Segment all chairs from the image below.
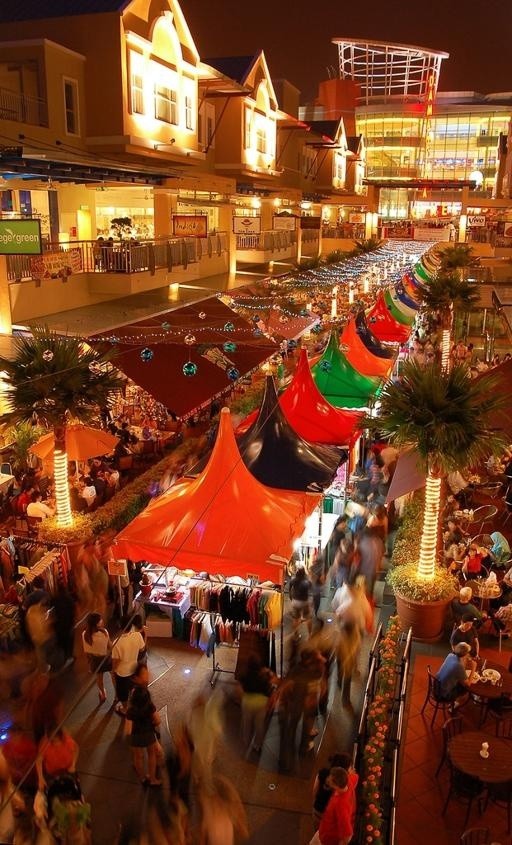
[6,403,135,531]
[419,484,512,845]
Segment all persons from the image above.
[1,313,512,845]
[93,237,140,272]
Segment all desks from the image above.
[134,577,199,640]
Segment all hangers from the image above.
[193,610,234,627]
[11,532,64,552]
